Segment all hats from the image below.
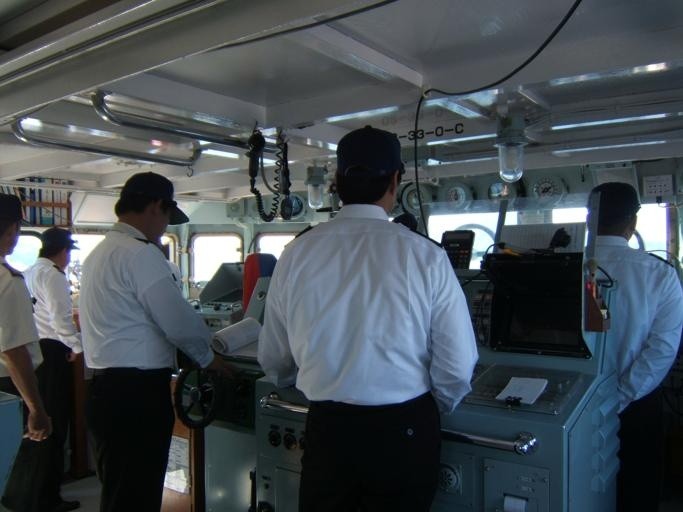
[40,226,79,250]
[588,183,640,219]
[0,194,32,227]
[121,171,189,225]
[338,125,404,174]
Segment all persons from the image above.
[584,182,683,512]
[0,193,54,511]
[257,126,478,509]
[2,229,84,511]
[79,172,222,512]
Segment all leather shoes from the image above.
[57,500,80,512]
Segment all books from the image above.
[214,316,262,361]
